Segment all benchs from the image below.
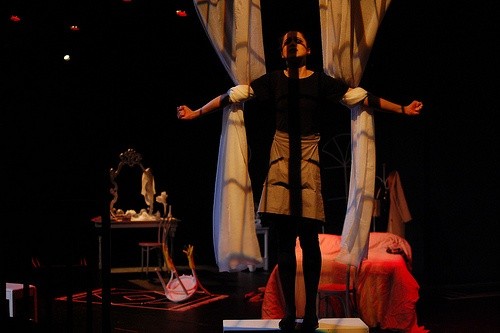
[223,317,368,333]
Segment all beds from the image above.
[262,233,416,328]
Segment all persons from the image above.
[176,27,422,331]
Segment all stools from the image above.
[6,283,39,324]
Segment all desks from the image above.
[92,216,178,276]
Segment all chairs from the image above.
[158,245,217,302]
[317,260,359,317]
[138,217,175,279]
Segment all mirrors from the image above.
[109,149,156,217]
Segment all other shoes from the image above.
[278,314,320,332]
